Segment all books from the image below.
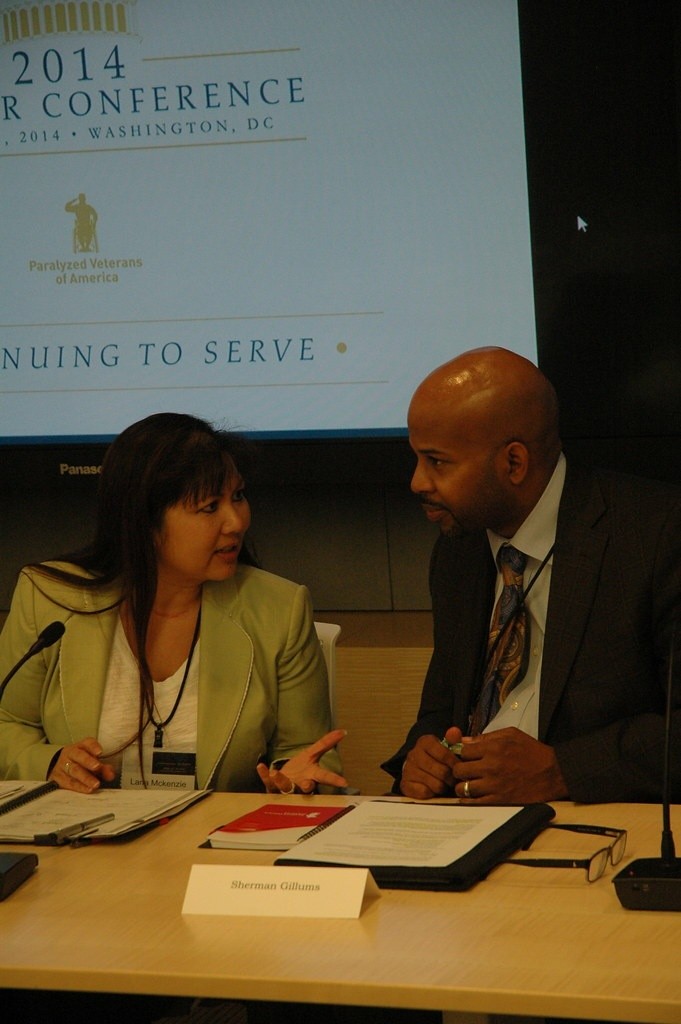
[207,804,355,851]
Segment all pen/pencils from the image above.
[47,813,115,845]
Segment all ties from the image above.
[467,543,529,739]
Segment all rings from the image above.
[64,761,73,774]
[280,782,295,795]
[464,781,471,797]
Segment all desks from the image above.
[0,782,681,1024]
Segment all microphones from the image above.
[0,622,66,702]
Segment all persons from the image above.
[0,413,351,795]
[381,346,681,805]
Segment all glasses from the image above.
[482,824,628,883]
